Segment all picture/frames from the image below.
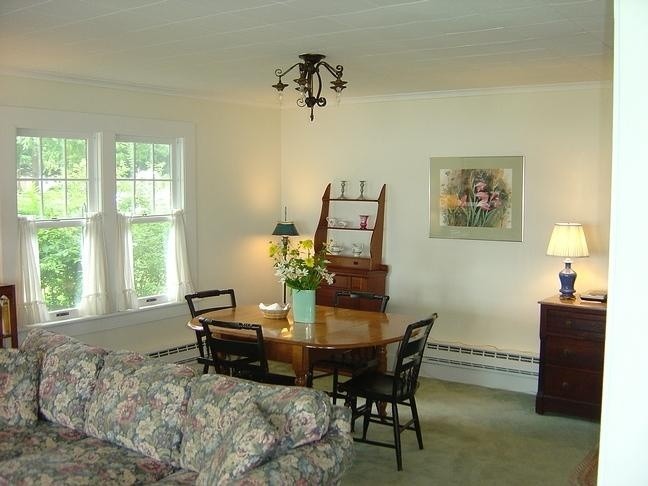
[429,156,522,243]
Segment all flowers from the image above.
[267,238,335,290]
[439,168,510,228]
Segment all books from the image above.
[579,289,607,301]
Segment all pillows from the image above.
[182,376,330,473]
[198,406,279,486]
[0,348,40,430]
[83,352,194,468]
[39,343,106,433]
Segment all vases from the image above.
[292,289,316,323]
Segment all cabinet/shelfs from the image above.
[306,182,387,377]
[535,294,606,424]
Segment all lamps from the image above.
[547,222,590,299]
[271,206,300,304]
[273,53,354,122]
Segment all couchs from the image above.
[0,332,353,485]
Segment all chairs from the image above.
[198,317,296,387]
[185,289,258,373]
[337,313,438,472]
[308,291,389,419]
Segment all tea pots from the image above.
[326,216,338,227]
